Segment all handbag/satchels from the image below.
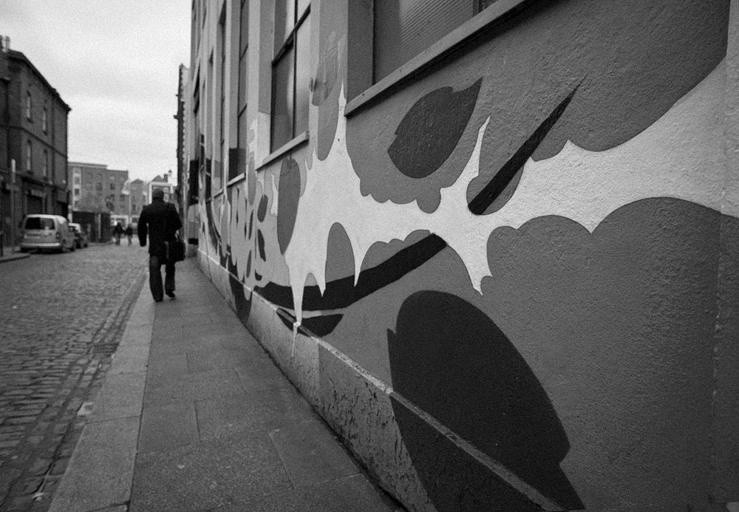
[164,235,188,263]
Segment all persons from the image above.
[112,222,125,245]
[137,188,183,303]
[125,225,133,245]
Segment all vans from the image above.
[18,214,77,254]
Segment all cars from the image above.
[68,223,88,249]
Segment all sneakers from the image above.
[154,297,164,303]
[165,289,176,300]
